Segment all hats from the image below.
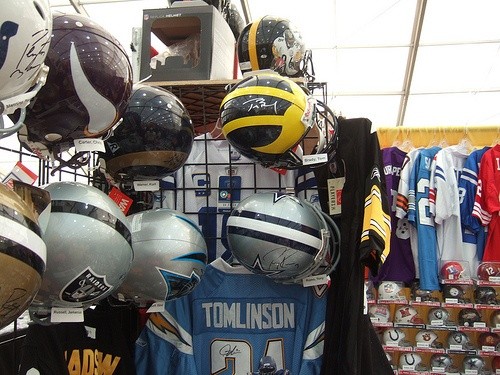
[218,71,340,171]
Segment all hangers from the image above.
[377,128,500,152]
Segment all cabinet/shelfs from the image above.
[367,277,500,375]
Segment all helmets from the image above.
[362,260,500,375]
[9,15,134,156]
[114,209,209,303]
[0,180,51,329]
[97,85,194,182]
[31,183,135,309]
[237,14,315,88]
[0,0,53,139]
[224,191,342,283]
